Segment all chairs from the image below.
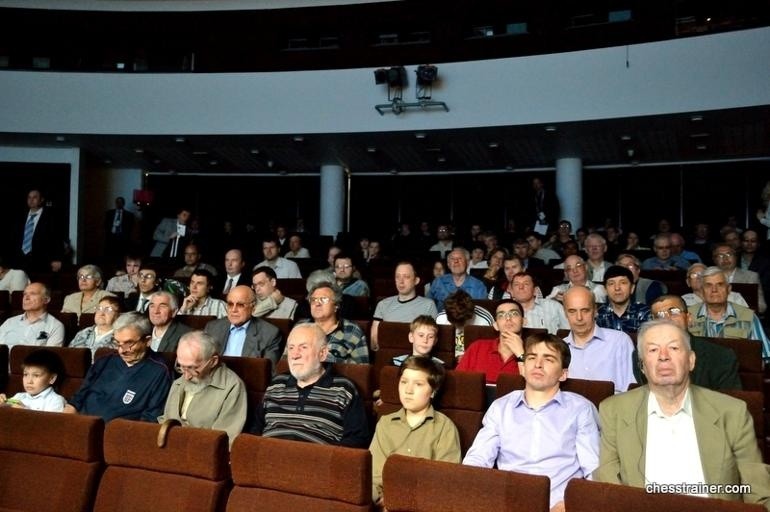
[290,257,316,276]
[218,356,272,436]
[274,360,374,443]
[292,317,370,342]
[0,405,105,512]
[276,278,309,315]
[627,381,765,464]
[526,268,564,297]
[376,364,486,463]
[93,346,182,386]
[373,321,457,369]
[93,417,227,510]
[641,268,681,296]
[693,336,765,392]
[494,372,617,413]
[337,293,377,317]
[223,431,373,512]
[175,314,217,332]
[10,289,62,315]
[6,344,91,402]
[12,310,77,347]
[463,324,548,354]
[381,452,550,511]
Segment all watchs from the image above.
[512,355,527,363]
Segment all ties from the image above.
[139,299,149,313]
[172,234,177,258]
[224,278,233,294]
[21,213,38,254]
[116,211,121,231]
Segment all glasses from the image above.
[174,358,211,377]
[110,340,146,350]
[335,264,352,268]
[566,264,586,271]
[226,302,254,308]
[743,238,758,244]
[252,280,269,290]
[311,298,337,305]
[438,229,449,232]
[79,275,98,280]
[559,224,570,228]
[94,306,119,313]
[715,253,733,259]
[655,308,684,318]
[655,245,672,251]
[496,310,524,317]
[138,273,157,280]
[621,263,638,268]
[688,272,701,280]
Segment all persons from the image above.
[632,295,741,388]
[645,237,684,273]
[492,253,543,298]
[437,292,496,359]
[425,259,444,299]
[736,233,767,283]
[421,223,433,240]
[553,221,582,259]
[287,234,309,260]
[358,240,370,258]
[98,198,135,260]
[251,240,303,281]
[621,230,648,252]
[427,248,489,315]
[2,264,31,304]
[613,256,667,307]
[6,191,61,280]
[509,240,544,277]
[142,293,194,354]
[70,312,174,421]
[503,274,567,330]
[370,261,438,353]
[323,252,370,299]
[545,253,606,302]
[157,330,250,450]
[106,258,143,300]
[277,283,369,369]
[274,227,289,254]
[687,222,713,263]
[576,227,588,251]
[118,268,162,319]
[67,297,120,352]
[217,222,237,256]
[0,284,64,349]
[395,221,412,243]
[597,319,770,510]
[523,174,559,239]
[460,336,602,511]
[366,358,462,510]
[606,226,619,249]
[211,249,251,297]
[581,234,612,282]
[685,267,769,368]
[250,323,365,451]
[463,243,487,281]
[667,232,699,269]
[364,244,383,274]
[650,219,669,241]
[596,267,649,335]
[374,314,446,408]
[206,286,279,379]
[151,212,190,257]
[528,234,559,265]
[60,266,115,316]
[422,262,445,298]
[485,236,509,255]
[454,300,529,386]
[718,229,744,265]
[307,246,361,295]
[2,352,66,410]
[468,225,481,240]
[710,243,765,313]
[178,269,227,321]
[556,286,635,394]
[478,248,507,296]
[430,226,454,256]
[250,268,298,320]
[175,246,221,289]
[681,262,750,309]
